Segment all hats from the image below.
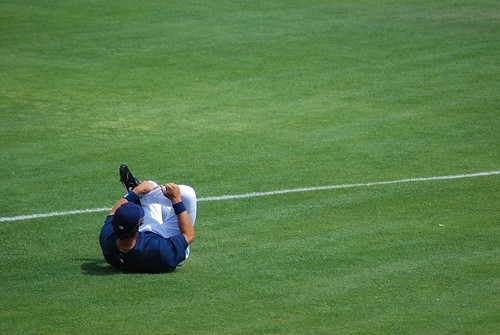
[114,202,145,227]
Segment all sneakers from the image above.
[120,164,142,199]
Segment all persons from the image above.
[99,164,197,274]
